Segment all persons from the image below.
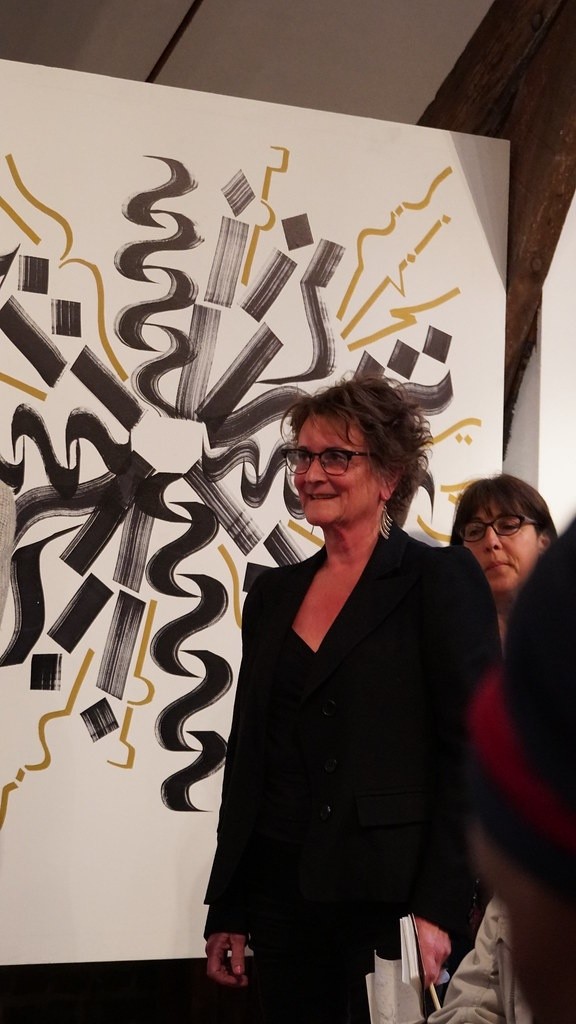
[427,472,576,1024]
[203,375,502,1024]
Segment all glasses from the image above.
[281,448,376,476]
[458,514,539,542]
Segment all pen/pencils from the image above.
[425,974,442,1012]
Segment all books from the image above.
[364,914,443,1024]
